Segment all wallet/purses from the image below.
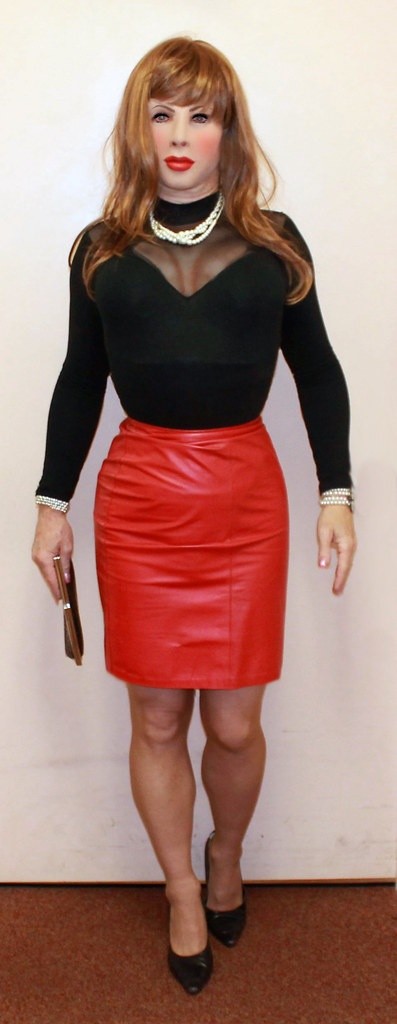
[53,556,84,666]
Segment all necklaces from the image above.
[149,193,226,245]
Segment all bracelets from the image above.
[319,488,354,512]
[35,494,69,514]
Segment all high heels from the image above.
[205,830,246,948]
[168,937,213,996]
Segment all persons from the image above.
[31,38,355,997]
